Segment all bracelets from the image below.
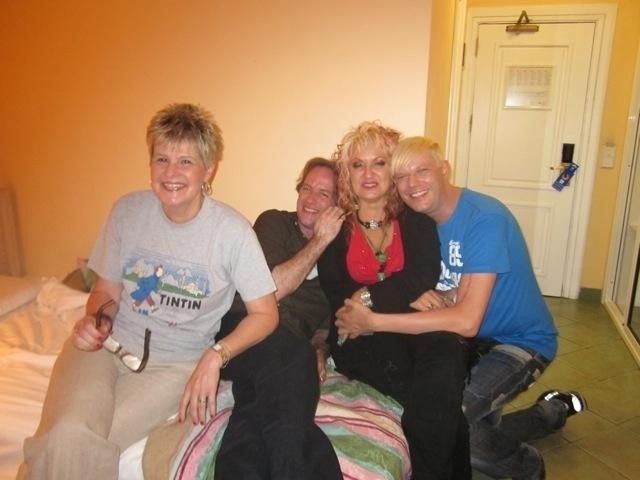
[209,342,233,368]
[358,284,374,311]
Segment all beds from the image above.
[0,182,413,480]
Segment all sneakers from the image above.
[536,388,588,419]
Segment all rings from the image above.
[428,304,433,309]
[443,295,448,301]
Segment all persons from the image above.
[14,101,280,480]
[334,132,590,480]
[317,117,472,480]
[215,153,347,480]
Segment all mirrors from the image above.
[626,246,640,344]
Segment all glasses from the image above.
[96,299,151,372]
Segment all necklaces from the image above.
[360,224,392,282]
[355,207,392,228]
